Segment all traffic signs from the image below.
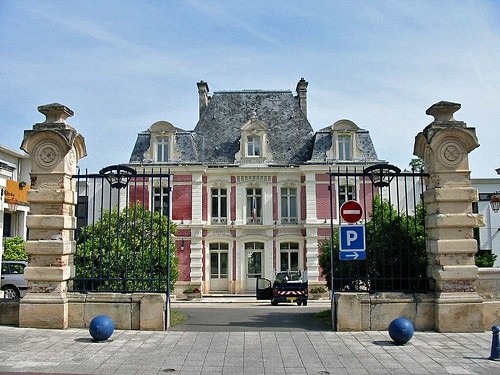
[338,225,367,260]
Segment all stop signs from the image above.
[339,200,363,223]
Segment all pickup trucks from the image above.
[255,270,309,307]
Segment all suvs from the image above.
[0,259,28,301]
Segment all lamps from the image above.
[181,238,184,251]
[487,191,500,212]
[19,182,26,188]
